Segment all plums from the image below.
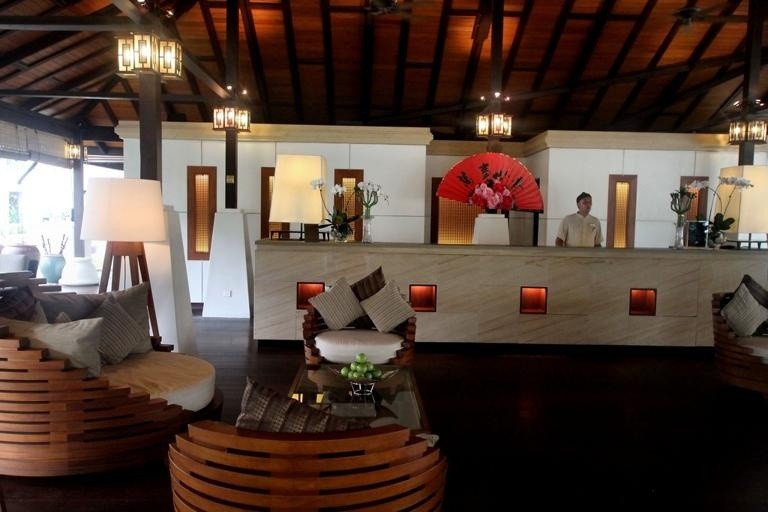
[339,353,383,379]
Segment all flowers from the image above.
[669,180,708,226]
[708,177,754,243]
[356,182,390,217]
[470,178,515,214]
[312,179,358,239]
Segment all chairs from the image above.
[0,272,223,476]
[170,419,448,512]
[302,296,416,365]
[711,291,766,393]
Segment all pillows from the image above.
[309,267,415,333]
[2,279,151,379]
[718,275,768,340]
[236,377,368,433]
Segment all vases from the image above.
[708,232,727,249]
[330,226,353,243]
[472,214,510,246]
[674,225,684,248]
[360,218,374,242]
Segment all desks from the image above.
[288,364,432,438]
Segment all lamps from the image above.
[80,179,165,340]
[729,98,767,145]
[476,91,516,139]
[116,0,183,80]
[213,84,251,132]
[269,154,328,242]
[63,142,88,162]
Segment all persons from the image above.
[555,192,604,247]
